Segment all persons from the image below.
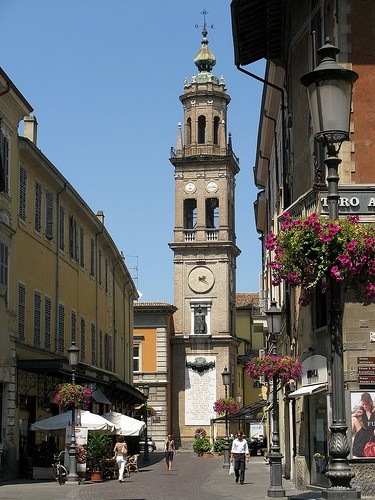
[113,435,127,482]
[234,433,248,470]
[230,430,249,485]
[352,392,375,458]
[164,435,175,471]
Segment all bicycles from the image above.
[52,451,68,486]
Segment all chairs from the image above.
[125,453,139,477]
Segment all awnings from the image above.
[19,355,149,404]
[288,381,327,397]
[100,411,147,436]
[30,409,122,434]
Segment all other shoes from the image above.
[235,480,239,484]
[118,479,124,483]
[240,481,244,485]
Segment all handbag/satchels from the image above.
[122,446,127,454]
[229,462,235,477]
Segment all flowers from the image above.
[51,383,92,409]
[213,398,240,415]
[245,355,304,386]
[265,210,375,305]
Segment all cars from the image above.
[138,436,155,453]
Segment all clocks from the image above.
[188,266,216,294]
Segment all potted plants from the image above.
[83,428,115,482]
[193,438,211,458]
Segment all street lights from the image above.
[263,297,286,498]
[139,384,150,461]
[66,338,80,482]
[220,365,231,469]
[300,34,358,492]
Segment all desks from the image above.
[100,457,114,463]
